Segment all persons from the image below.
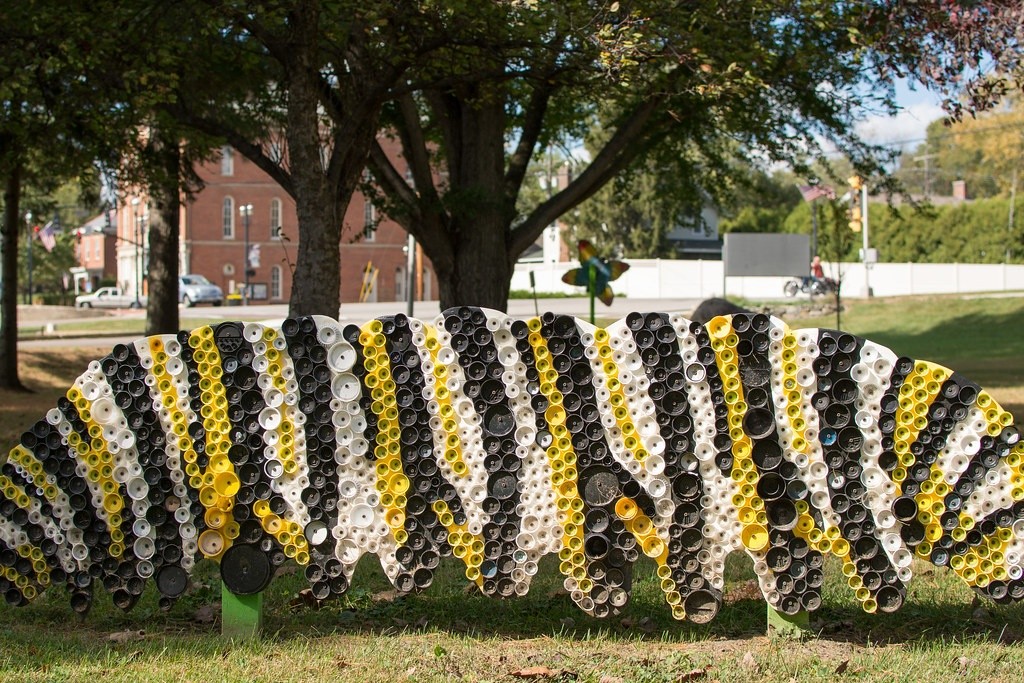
[810,256,825,290]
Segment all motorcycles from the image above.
[783,274,838,299]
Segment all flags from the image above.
[38,220,56,253]
[795,183,835,202]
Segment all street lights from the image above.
[131,195,149,307]
[25,208,32,305]
[238,204,255,306]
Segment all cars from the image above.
[74,285,148,310]
[179,274,224,307]
